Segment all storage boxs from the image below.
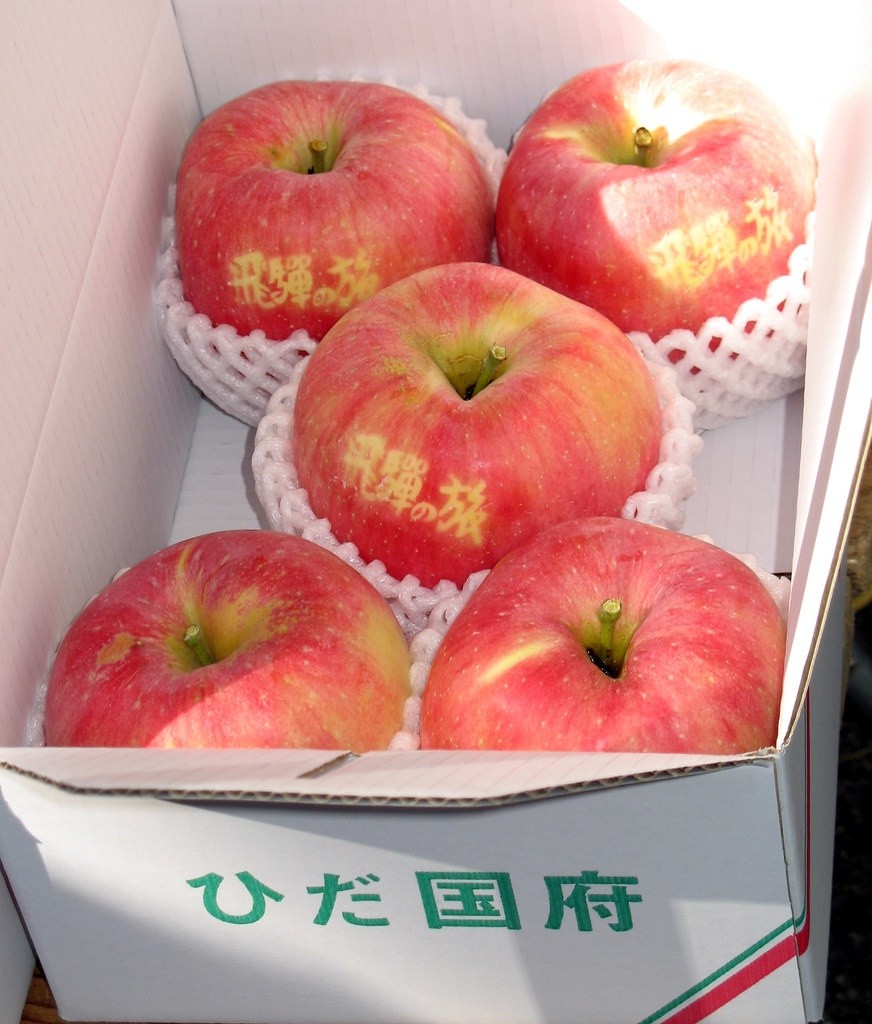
[0,0,872,1024]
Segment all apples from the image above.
[45,60,815,755]
